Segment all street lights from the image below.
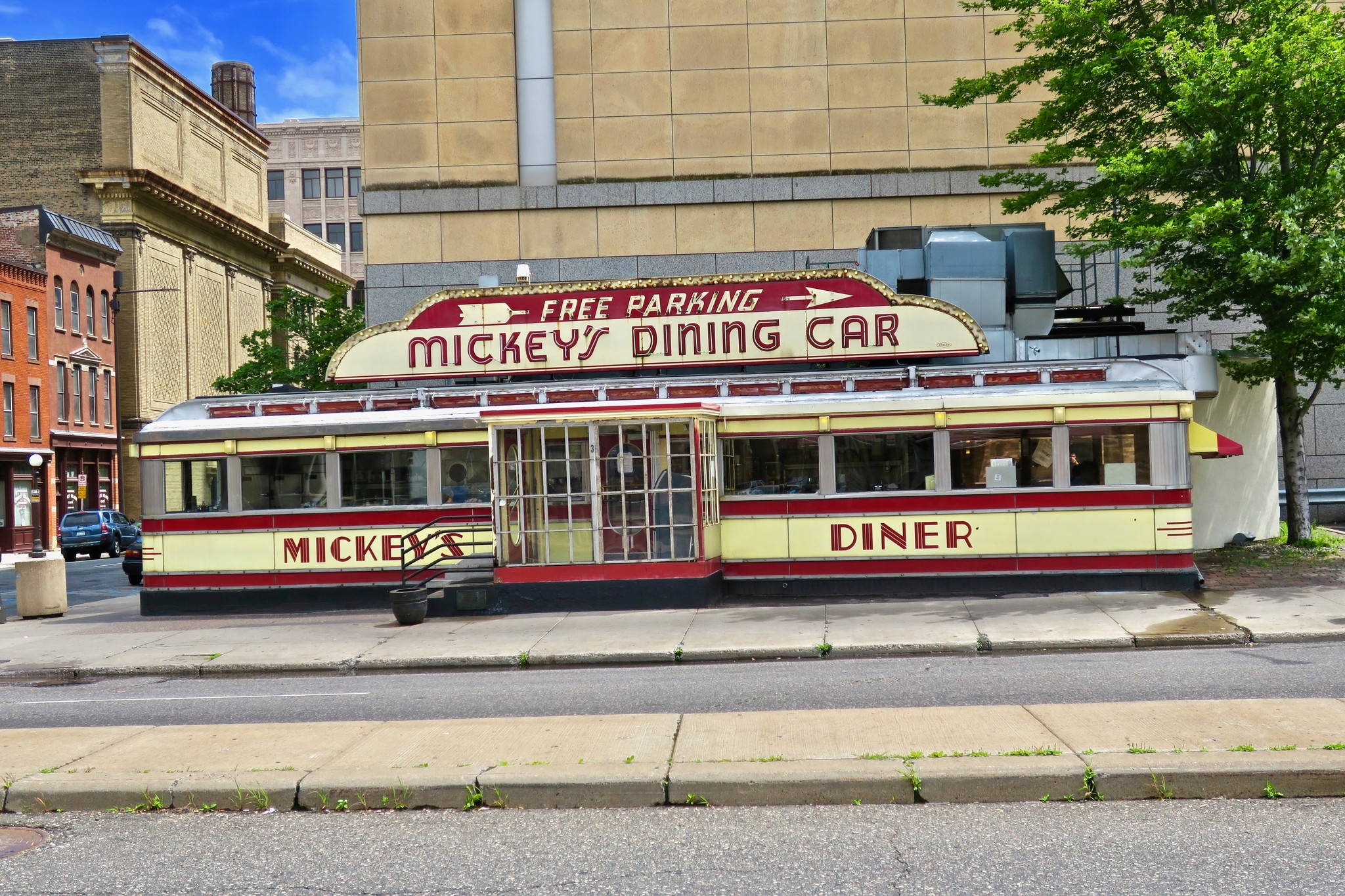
[28,454,47,558]
[112,288,181,514]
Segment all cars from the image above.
[122,518,143,586]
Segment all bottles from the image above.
[202,501,205,512]
[362,498,366,506]
[206,504,208,512]
[209,504,212,512]
[374,495,377,505]
[187,509,191,512]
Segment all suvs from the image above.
[57,508,142,562]
